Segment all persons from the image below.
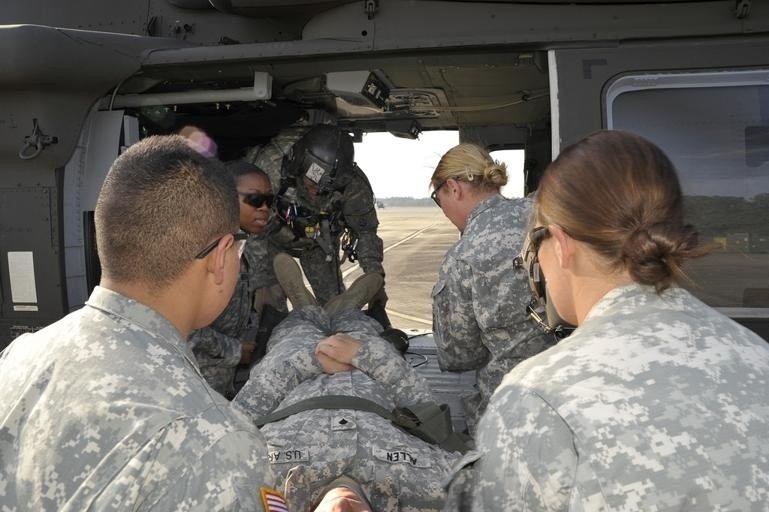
[427,143,565,439]
[229,252,464,512]
[472,125,769,512]
[0,136,272,512]
[189,163,281,393]
[247,118,387,310]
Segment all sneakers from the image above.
[274,252,316,309]
[323,271,383,315]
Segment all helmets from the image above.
[288,125,354,192]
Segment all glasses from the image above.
[195,226,251,259]
[236,192,275,209]
[430,180,450,208]
[530,225,551,253]
